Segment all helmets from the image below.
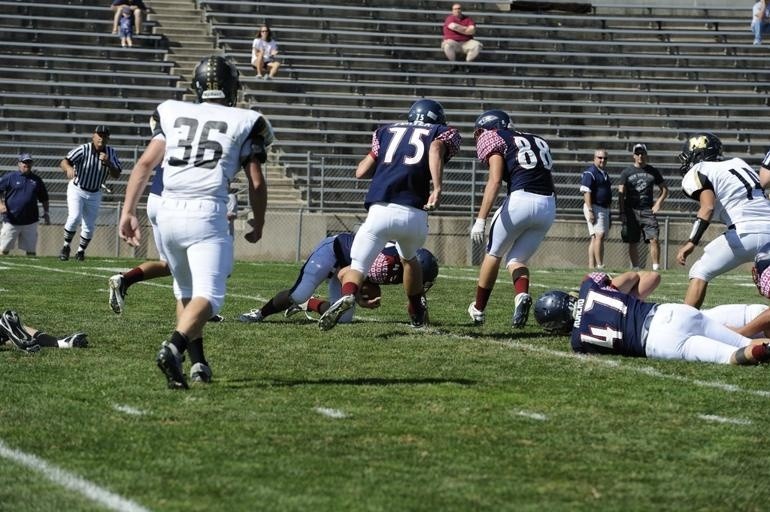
[681,132,725,169]
[473,109,515,136]
[189,54,242,103]
[531,290,578,334]
[416,246,438,287]
[405,98,447,128]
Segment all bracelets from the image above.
[44,211,50,216]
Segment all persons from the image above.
[119,8,133,48]
[441,4,483,73]
[751,0,770,45]
[536,270,770,365]
[251,26,280,79]
[59,125,122,261]
[677,132,770,308]
[317,100,462,331]
[468,111,557,328]
[110,0,146,35]
[241,233,438,324]
[0,153,51,256]
[0,311,90,354]
[109,160,237,324]
[117,55,273,390]
[580,149,612,269]
[618,143,667,271]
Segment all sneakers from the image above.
[155,339,189,391]
[107,274,128,315]
[0,310,41,352]
[467,298,484,326]
[61,332,89,348]
[317,291,355,333]
[73,251,86,261]
[510,292,535,330]
[283,294,314,319]
[239,308,265,323]
[206,314,225,322]
[59,246,70,260]
[189,362,213,384]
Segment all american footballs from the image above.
[360,283,381,305]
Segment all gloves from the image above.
[468,217,488,244]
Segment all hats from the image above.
[94,125,111,135]
[632,142,648,155]
[17,153,34,163]
